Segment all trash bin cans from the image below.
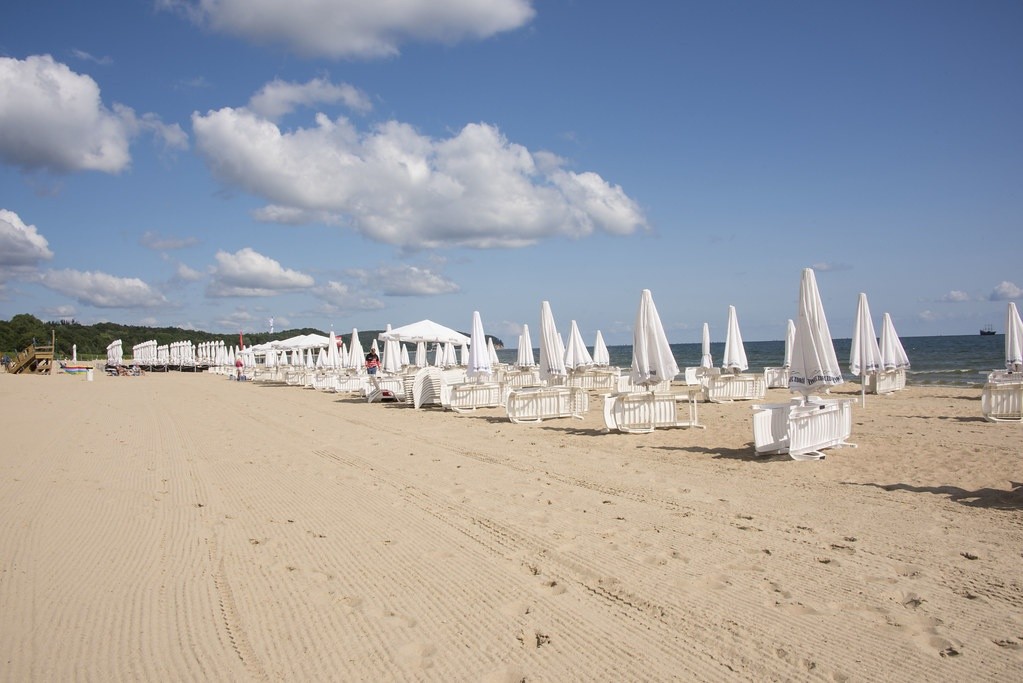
[86,367,93,381]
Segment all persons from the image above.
[364,346,381,375]
[236,358,244,376]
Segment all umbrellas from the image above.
[782,319,797,373]
[564,319,594,371]
[401,344,410,366]
[517,323,535,367]
[628,289,680,391]
[105,339,225,367]
[538,301,568,381]
[215,344,315,368]
[372,339,381,362]
[558,332,566,360]
[237,333,342,356]
[416,342,470,368]
[879,312,911,375]
[720,304,749,377]
[466,311,493,378]
[1005,302,1023,372]
[788,268,845,403]
[487,337,499,366]
[592,330,610,367]
[378,321,472,346]
[700,322,714,378]
[317,328,365,371]
[380,324,402,373]
[848,292,885,409]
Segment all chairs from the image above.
[105,363,1023,460]
